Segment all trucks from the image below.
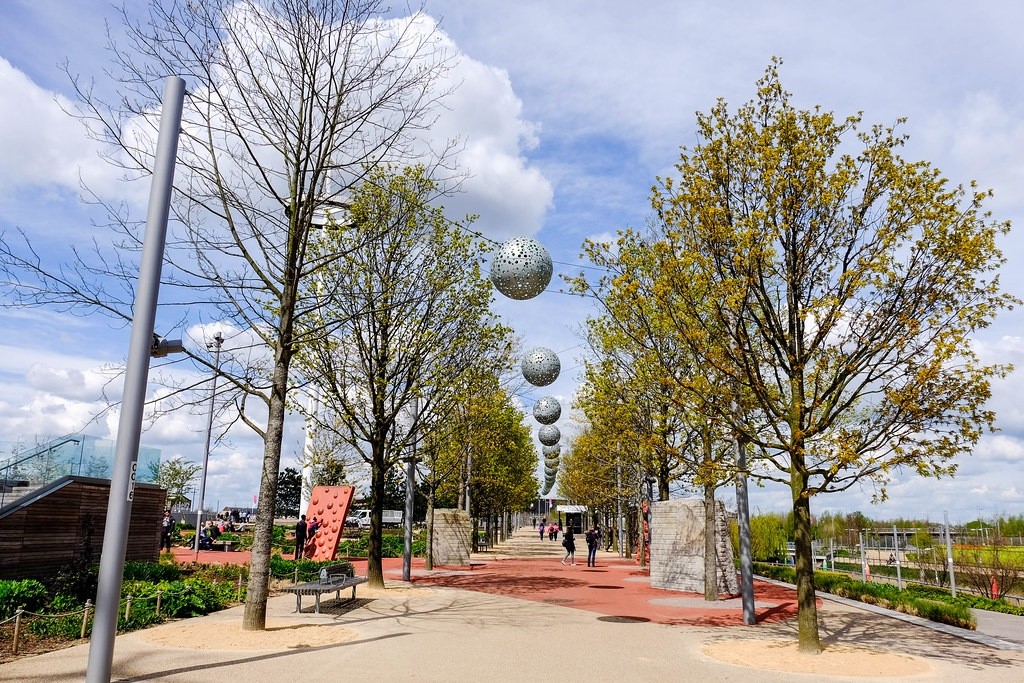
[347,510,403,529]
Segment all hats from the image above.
[313,517,317,520]
[590,526,594,530]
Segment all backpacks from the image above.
[549,527,554,532]
[562,540,566,547]
[540,526,543,530]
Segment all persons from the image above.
[585,527,602,568]
[160,510,175,553]
[217,509,250,532]
[532,517,536,529]
[542,517,547,525]
[569,519,573,526]
[185,521,219,551]
[606,526,614,552]
[295,515,321,561]
[538,523,559,542]
[561,527,576,566]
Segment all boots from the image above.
[588,556,590,567]
[592,556,594,567]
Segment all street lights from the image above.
[194,331,225,552]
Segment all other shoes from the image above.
[571,564,576,566]
[561,560,565,565]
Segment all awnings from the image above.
[556,505,588,527]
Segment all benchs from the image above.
[278,576,369,614]
[187,539,239,553]
[477,543,490,552]
